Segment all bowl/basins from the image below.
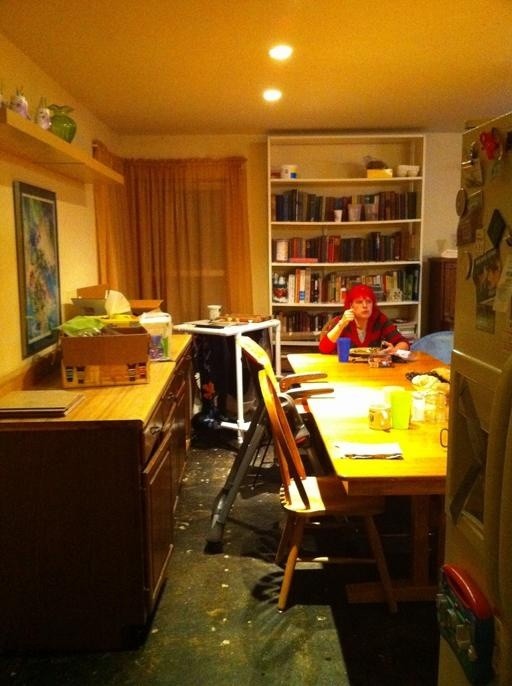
[411,374,441,393]
[437,381,449,396]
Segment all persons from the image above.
[319,284,410,356]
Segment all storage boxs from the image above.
[70,284,165,316]
[59,326,153,390]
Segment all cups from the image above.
[387,390,413,431]
[207,305,222,319]
[423,392,446,426]
[368,404,391,431]
[333,209,343,222]
[336,337,351,362]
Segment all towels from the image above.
[334,441,404,460]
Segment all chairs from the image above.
[257,368,399,611]
[204,335,336,544]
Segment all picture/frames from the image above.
[444,350,507,558]
[14,180,62,360]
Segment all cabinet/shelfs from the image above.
[267,133,426,359]
[171,317,282,451]
[1,332,193,644]
[428,257,458,332]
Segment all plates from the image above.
[348,347,384,357]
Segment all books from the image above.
[0,390,86,418]
[271,188,419,340]
